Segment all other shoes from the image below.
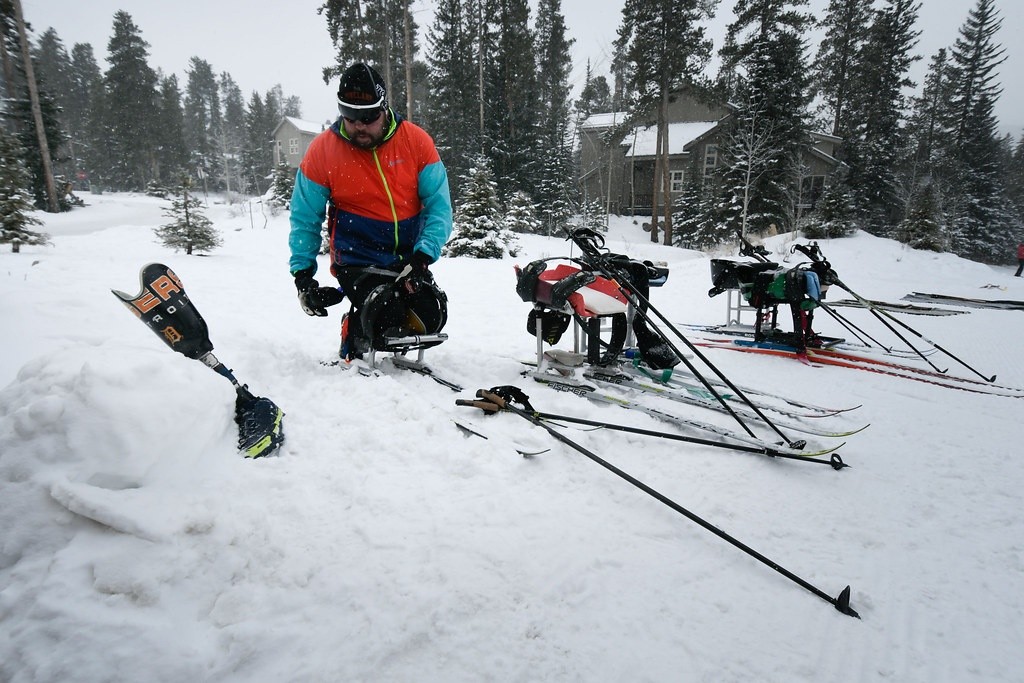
[234,395,285,459]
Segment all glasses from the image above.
[336,104,382,126]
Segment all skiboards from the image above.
[673,322,1024,399]
[519,354,873,458]
[317,349,612,457]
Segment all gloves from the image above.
[394,254,432,293]
[291,272,344,317]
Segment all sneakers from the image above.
[337,312,365,365]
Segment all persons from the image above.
[1015,241,1024,277]
[288,63,453,364]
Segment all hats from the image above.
[337,63,386,106]
